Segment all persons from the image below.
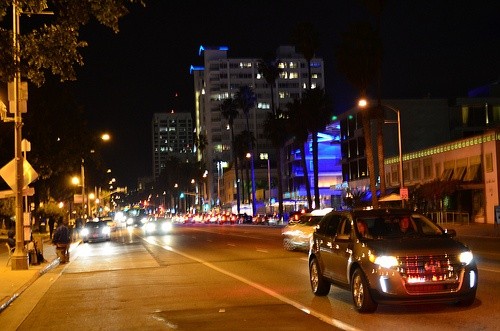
[391,217,414,235]
[7,231,48,265]
[356,220,375,238]
[48,215,55,239]
[52,217,70,264]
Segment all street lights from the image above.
[358,93,404,208]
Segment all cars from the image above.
[77,205,293,244]
[282,206,335,250]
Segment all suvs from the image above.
[309,208,479,311]
